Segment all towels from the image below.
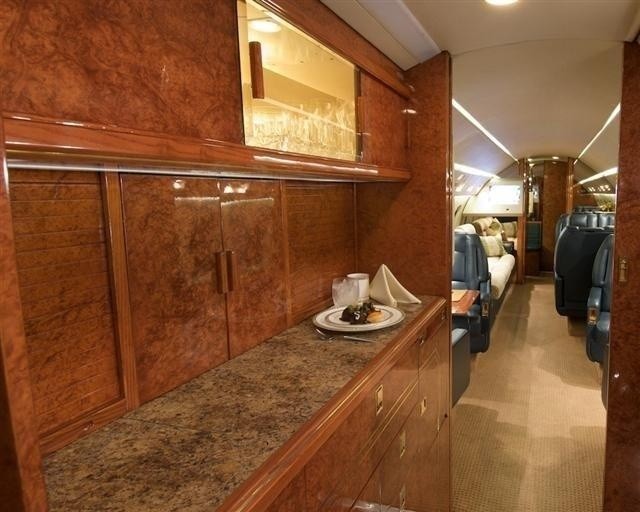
[368,264,422,308]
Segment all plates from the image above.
[312,305,406,332]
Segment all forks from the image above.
[313,328,376,343]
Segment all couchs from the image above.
[452,234,490,352]
[471,217,518,255]
[456,225,515,317]
[567,213,614,227]
[553,226,614,316]
[586,235,614,361]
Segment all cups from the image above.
[347,273,369,300]
[331,277,359,307]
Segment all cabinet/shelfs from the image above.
[1,0,454,511]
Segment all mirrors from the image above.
[462,214,518,255]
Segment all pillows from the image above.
[487,230,503,241]
[490,218,503,230]
[480,235,506,257]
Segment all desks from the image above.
[452,290,479,313]
